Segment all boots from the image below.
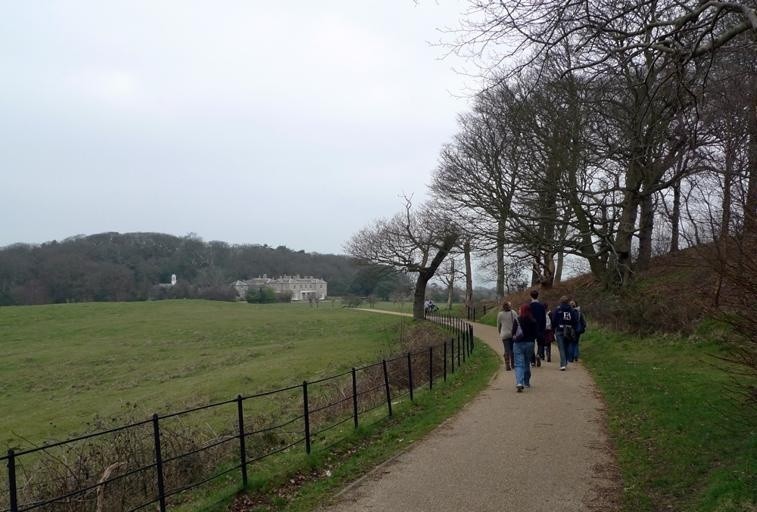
[504,351,514,370]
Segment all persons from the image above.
[496,289,585,371]
[511,304,537,392]
[424,298,433,319]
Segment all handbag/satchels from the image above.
[546,311,553,330]
[577,312,585,333]
[512,317,524,341]
[564,325,576,342]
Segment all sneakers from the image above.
[531,352,578,370]
[516,383,530,392]
